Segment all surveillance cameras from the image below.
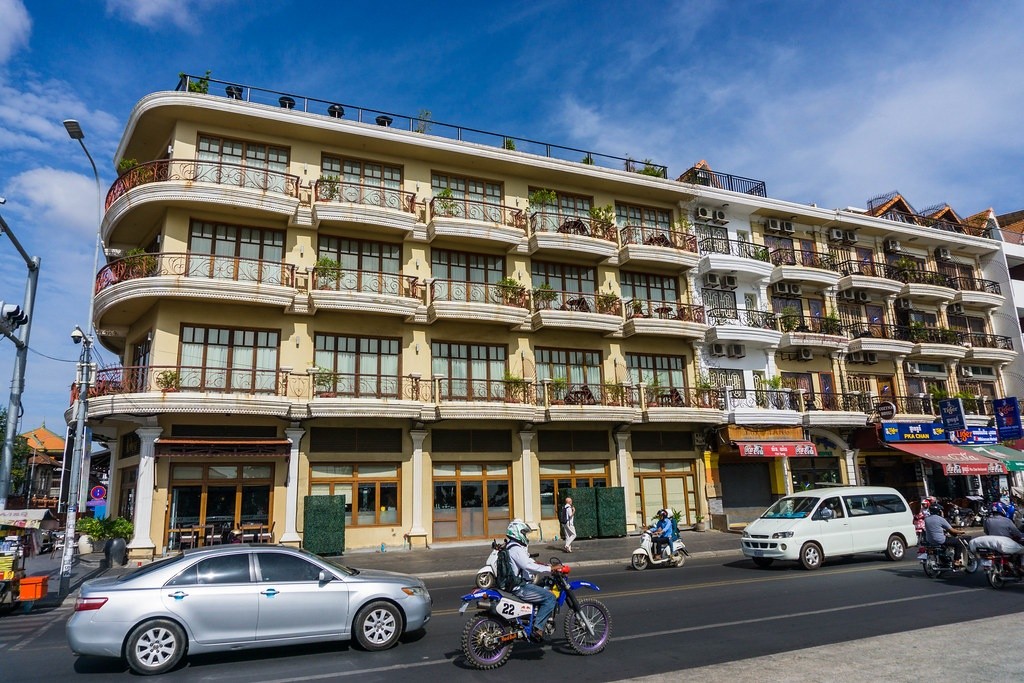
[71,330,82,343]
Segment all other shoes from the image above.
[564,544,572,553]
[1014,566,1024,576]
[954,561,965,567]
[670,554,674,560]
[652,552,661,561]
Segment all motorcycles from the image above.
[916,531,980,579]
[976,547,1024,588]
[461,556,613,671]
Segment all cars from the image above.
[0,529,79,558]
[65,542,433,676]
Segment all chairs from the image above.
[177,524,196,551]
[241,524,262,544]
[206,525,223,547]
[258,521,276,543]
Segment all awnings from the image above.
[886,443,1024,476]
[732,440,819,458]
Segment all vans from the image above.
[740,486,918,571]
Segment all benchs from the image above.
[127,547,155,562]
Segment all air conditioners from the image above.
[728,344,746,357]
[779,222,795,235]
[843,231,859,245]
[849,352,864,363]
[884,239,902,253]
[854,291,872,304]
[721,275,738,290]
[713,211,730,224]
[934,248,951,261]
[948,303,965,316]
[839,289,855,301]
[896,298,913,310]
[710,343,726,358]
[829,229,843,241]
[863,352,879,364]
[958,366,974,377]
[694,207,714,220]
[797,348,814,360]
[904,361,920,375]
[703,271,720,285]
[773,282,789,295]
[765,219,781,234]
[786,284,803,297]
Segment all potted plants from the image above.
[312,255,345,291]
[75,514,134,553]
[643,373,665,408]
[548,377,569,405]
[309,364,347,399]
[587,203,617,241]
[631,297,645,318]
[156,369,183,392]
[494,276,527,307]
[123,248,159,278]
[695,383,713,408]
[499,368,529,404]
[533,281,558,310]
[317,172,341,202]
[601,380,625,406]
[528,188,558,232]
[436,187,461,217]
[597,290,620,315]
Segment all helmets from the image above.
[657,509,667,521]
[665,509,674,518]
[990,501,1006,517]
[921,499,930,508]
[929,503,942,515]
[506,521,532,546]
[926,496,936,505]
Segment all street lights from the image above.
[61,118,102,342]
[57,324,91,598]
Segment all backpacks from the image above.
[496,542,523,593]
[558,505,572,524]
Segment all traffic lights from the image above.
[0,298,29,335]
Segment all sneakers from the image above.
[532,627,551,643]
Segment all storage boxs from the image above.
[0,555,19,573]
[10,546,18,551]
[4,571,15,579]
[5,535,19,540]
[5,552,12,556]
[19,576,49,599]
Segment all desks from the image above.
[566,300,584,311]
[657,394,675,407]
[571,390,590,405]
[240,525,270,531]
[169,528,203,532]
[654,307,673,319]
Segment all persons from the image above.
[818,502,832,519]
[920,499,931,517]
[926,496,944,517]
[500,519,564,646]
[665,509,680,559]
[562,498,577,553]
[984,502,1024,573]
[925,504,966,567]
[645,510,673,559]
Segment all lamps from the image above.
[296,336,300,346]
[416,344,420,353]
[304,162,308,171]
[517,195,520,202]
[167,144,173,153]
[300,245,304,254]
[148,331,153,341]
[415,258,420,266]
[610,279,613,287]
[522,350,525,359]
[157,234,161,243]
[518,269,522,277]
[417,180,420,187]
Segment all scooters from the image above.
[632,529,690,572]
[914,499,992,541]
[474,539,501,590]
